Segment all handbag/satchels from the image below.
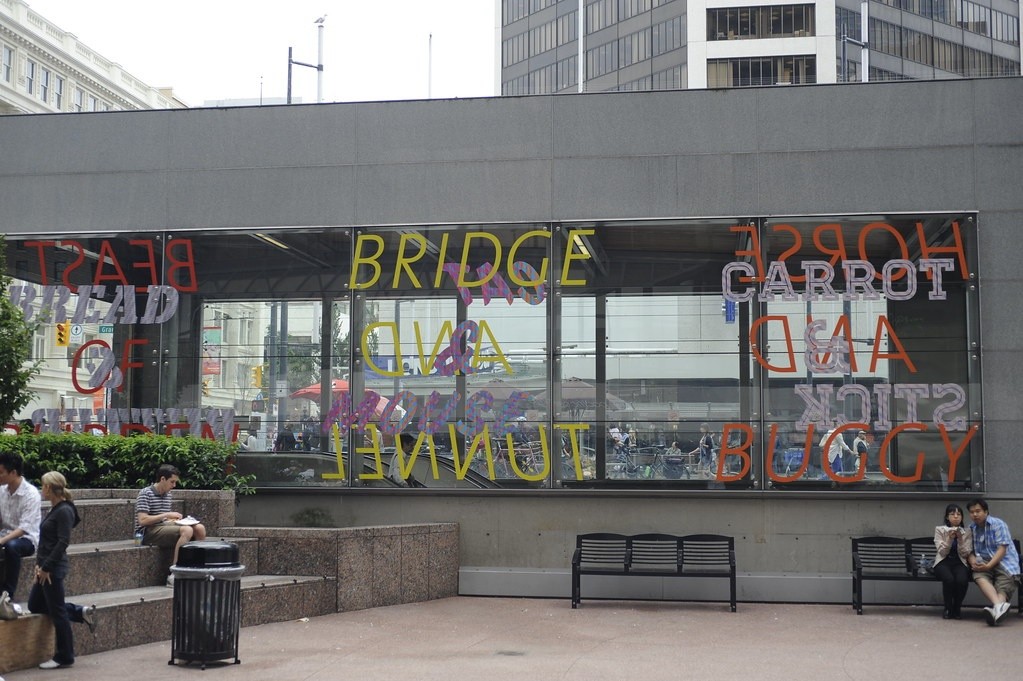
[918,552,931,575]
[709,449,717,462]
[0,591,18,620]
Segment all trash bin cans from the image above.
[167,542,246,668]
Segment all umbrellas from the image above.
[467,378,531,417]
[289,378,368,406]
[535,377,625,434]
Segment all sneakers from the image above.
[983,606,995,626]
[993,601,1011,623]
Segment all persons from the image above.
[387,433,417,487]
[689,423,713,479]
[0,451,41,620]
[606,424,643,454]
[768,425,783,475]
[967,498,1021,625]
[295,406,384,450]
[665,441,682,455]
[853,431,869,478]
[239,430,257,451]
[27,471,96,669]
[933,504,976,619]
[134,464,206,588]
[818,421,857,474]
[561,436,570,459]
[276,420,295,451]
[505,417,533,473]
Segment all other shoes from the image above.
[864,476,869,479]
[81,605,98,633]
[165,575,174,587]
[943,609,951,619]
[952,612,961,620]
[39,658,74,670]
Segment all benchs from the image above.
[850,537,1023,615]
[572,533,737,612]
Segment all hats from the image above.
[768,425,773,428]
[753,424,758,428]
[859,430,867,434]
